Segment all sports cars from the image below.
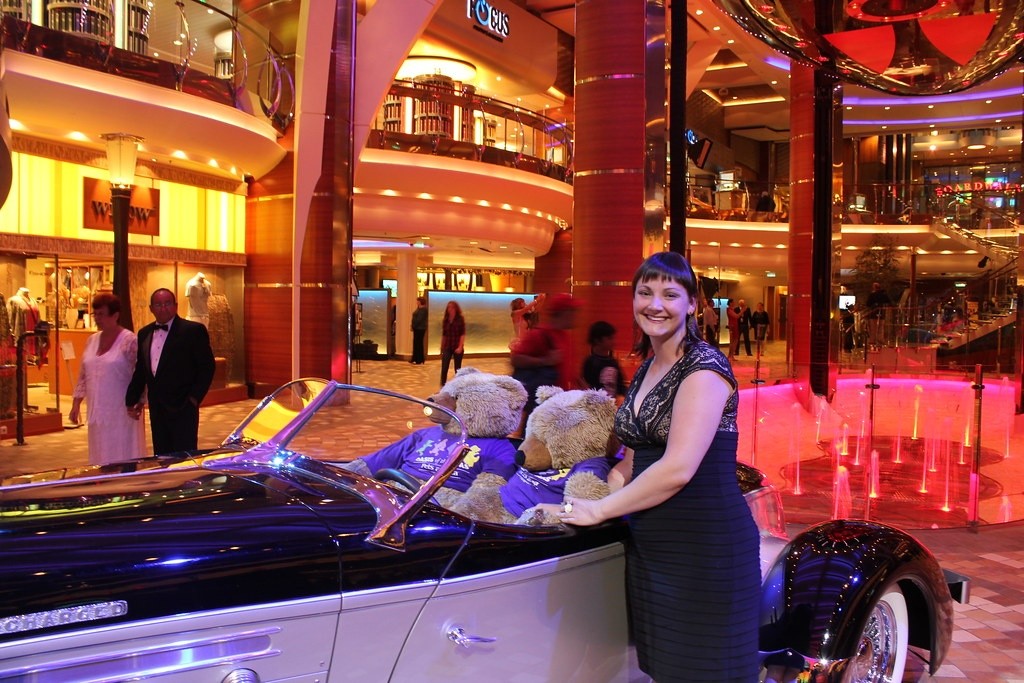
[1,372,973,683]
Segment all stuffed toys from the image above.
[457,386,624,527]
[340,366,528,518]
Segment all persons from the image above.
[557,252,762,683]
[439,301,466,387]
[724,298,769,361]
[756,191,776,213]
[703,300,720,350]
[125,288,216,459]
[8,287,41,353]
[184,272,211,328]
[69,293,146,467]
[409,295,429,365]
[509,292,631,436]
[844,304,857,353]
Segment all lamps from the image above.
[685,137,714,172]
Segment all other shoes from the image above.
[746,353,752,356]
[734,352,739,356]
[761,351,764,356]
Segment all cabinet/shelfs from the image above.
[44,259,148,392]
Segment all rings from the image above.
[565,504,572,513]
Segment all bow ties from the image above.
[152,324,169,332]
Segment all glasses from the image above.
[152,301,176,310]
[89,311,111,318]
